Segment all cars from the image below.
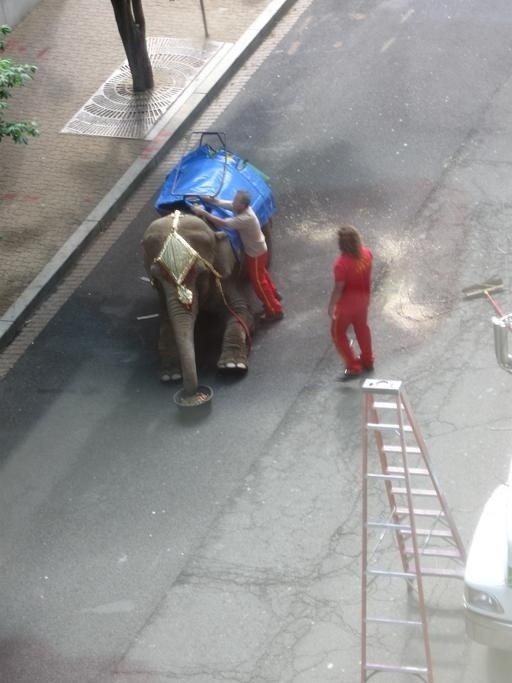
[462,480,511,650]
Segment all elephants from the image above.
[139,148,273,398]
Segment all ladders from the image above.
[361,378,467,683]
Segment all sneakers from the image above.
[259,292,283,321]
[339,356,374,379]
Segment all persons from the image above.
[191,191,284,322]
[328,226,373,380]
